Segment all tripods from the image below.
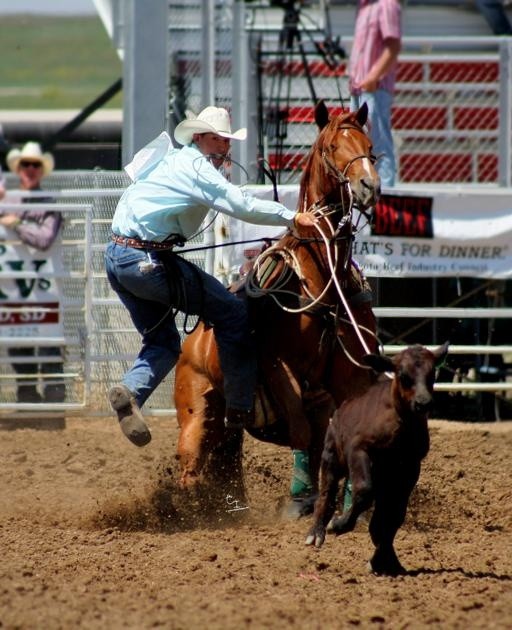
[264,11,333,183]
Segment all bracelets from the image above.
[11,220,21,231]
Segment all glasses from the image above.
[20,161,42,169]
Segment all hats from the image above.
[173,105,248,148]
[6,141,55,177]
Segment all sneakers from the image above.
[223,406,245,430]
[108,384,152,447]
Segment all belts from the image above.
[111,231,174,252]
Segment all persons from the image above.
[104,106,320,446]
[2,140,68,403]
[347,1,404,187]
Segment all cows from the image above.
[305,340,448,577]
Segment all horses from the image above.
[175,98,379,515]
